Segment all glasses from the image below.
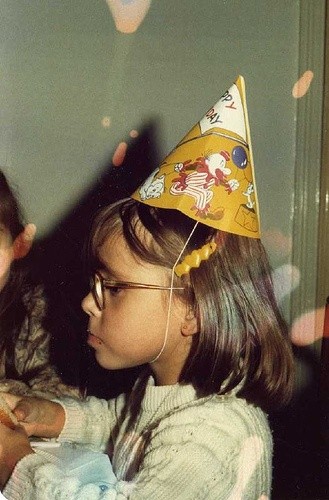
[90,270,187,311]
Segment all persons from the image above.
[0,75,295,500]
[0,169,85,412]
